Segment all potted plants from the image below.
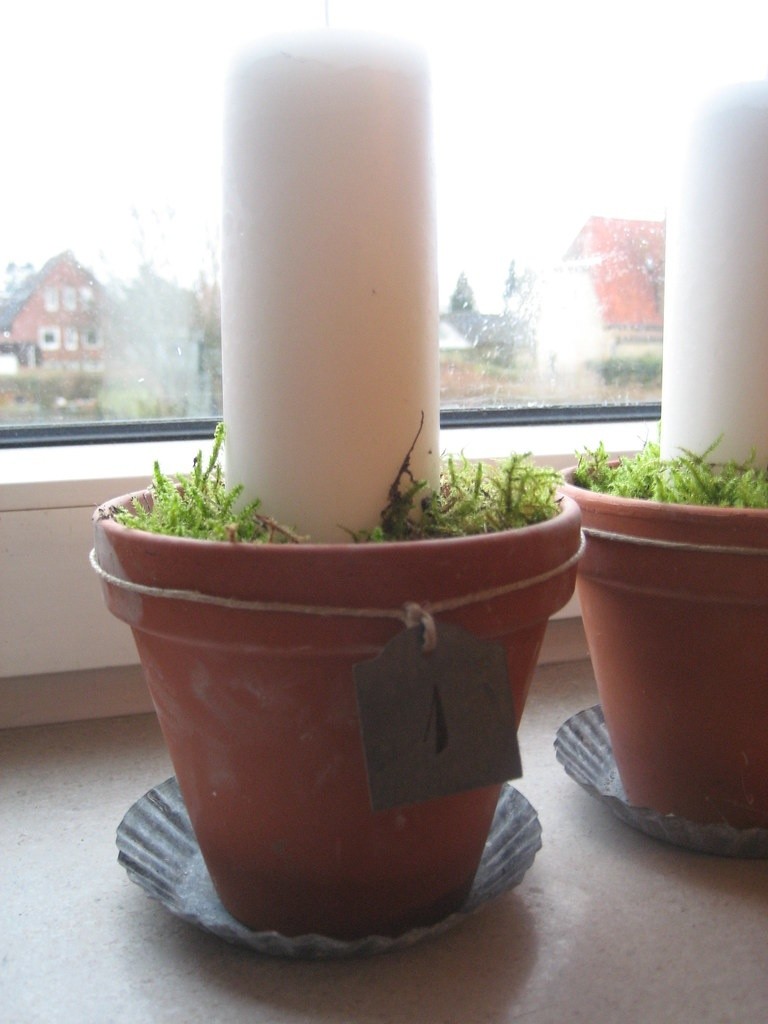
[559,419,768,830]
[84,407,588,933]
